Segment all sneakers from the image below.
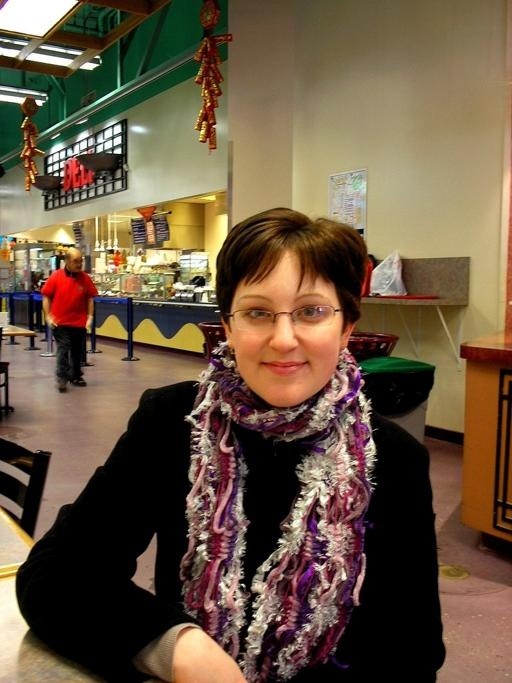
[70,375,87,387]
[58,381,67,393]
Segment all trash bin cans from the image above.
[359,356,435,445]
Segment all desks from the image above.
[0,510,150,683]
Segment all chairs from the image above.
[0,326,15,416]
[0,435,53,538]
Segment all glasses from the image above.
[225,304,342,331]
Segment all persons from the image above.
[42,245,99,395]
[12,205,448,682]
